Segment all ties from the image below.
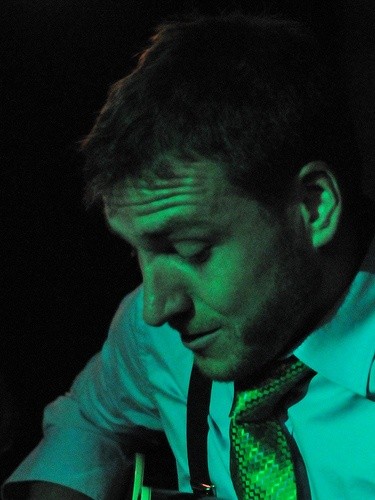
[230,354,319,500]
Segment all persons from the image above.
[2,12,374,500]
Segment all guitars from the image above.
[121,444,199,500]
